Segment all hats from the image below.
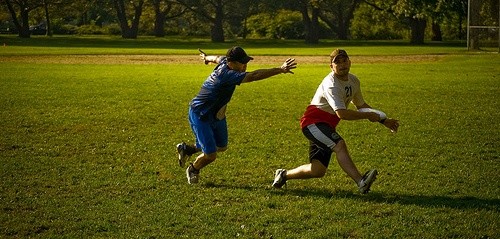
[226,46,254,64]
[330,49,349,62]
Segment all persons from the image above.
[271,48,400,194]
[177,46,298,185]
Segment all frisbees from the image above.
[359,108,386,120]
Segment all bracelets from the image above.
[379,117,386,123]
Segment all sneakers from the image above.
[177,141,192,168]
[186,163,199,184]
[272,168,286,188]
[361,170,377,194]
